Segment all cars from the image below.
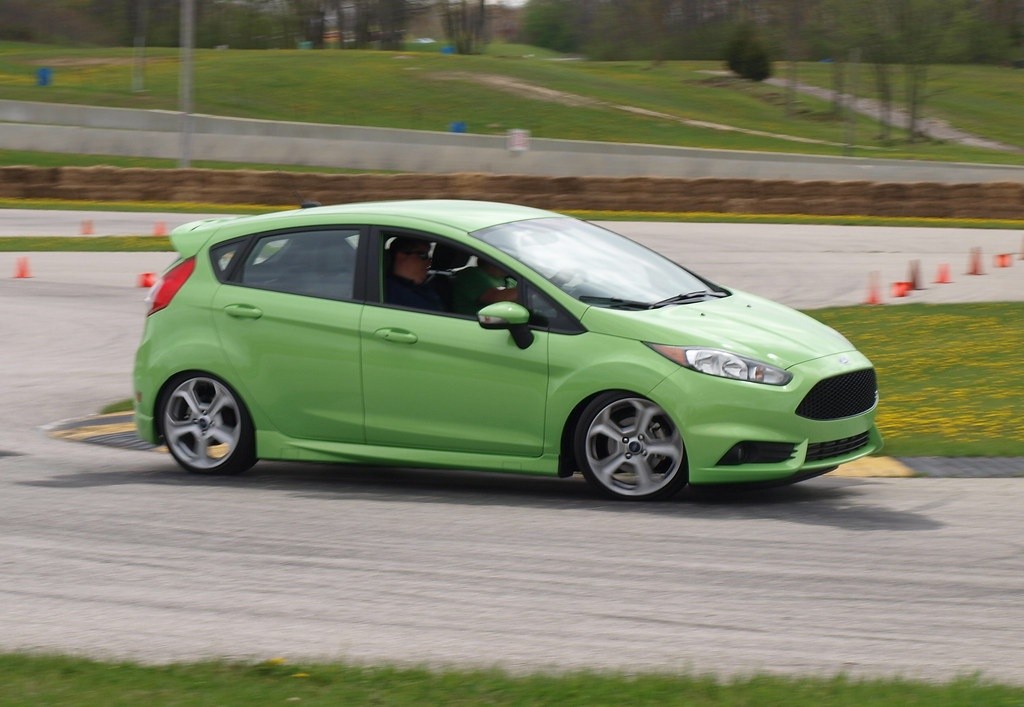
[132,199,884,505]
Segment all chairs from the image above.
[426,240,470,310]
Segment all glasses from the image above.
[401,252,432,261]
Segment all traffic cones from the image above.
[13,254,33,280]
[82,219,99,235]
[155,222,165,237]
[139,272,157,287]
[865,245,1012,308]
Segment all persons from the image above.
[381,234,453,315]
[456,253,575,318]
